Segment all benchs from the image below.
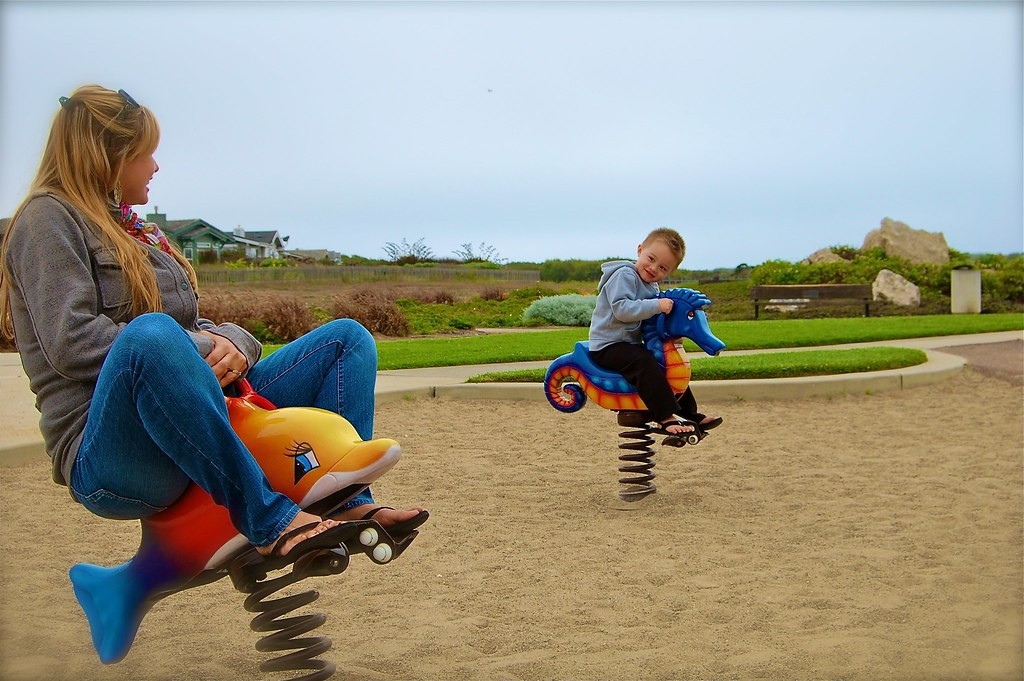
[750,285,884,320]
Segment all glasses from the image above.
[96,88,140,140]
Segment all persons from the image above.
[0,86,432,579]
[588,227,723,437]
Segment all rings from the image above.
[227,368,241,376]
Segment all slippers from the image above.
[697,417,723,431]
[650,418,697,435]
[360,507,429,537]
[262,520,358,566]
